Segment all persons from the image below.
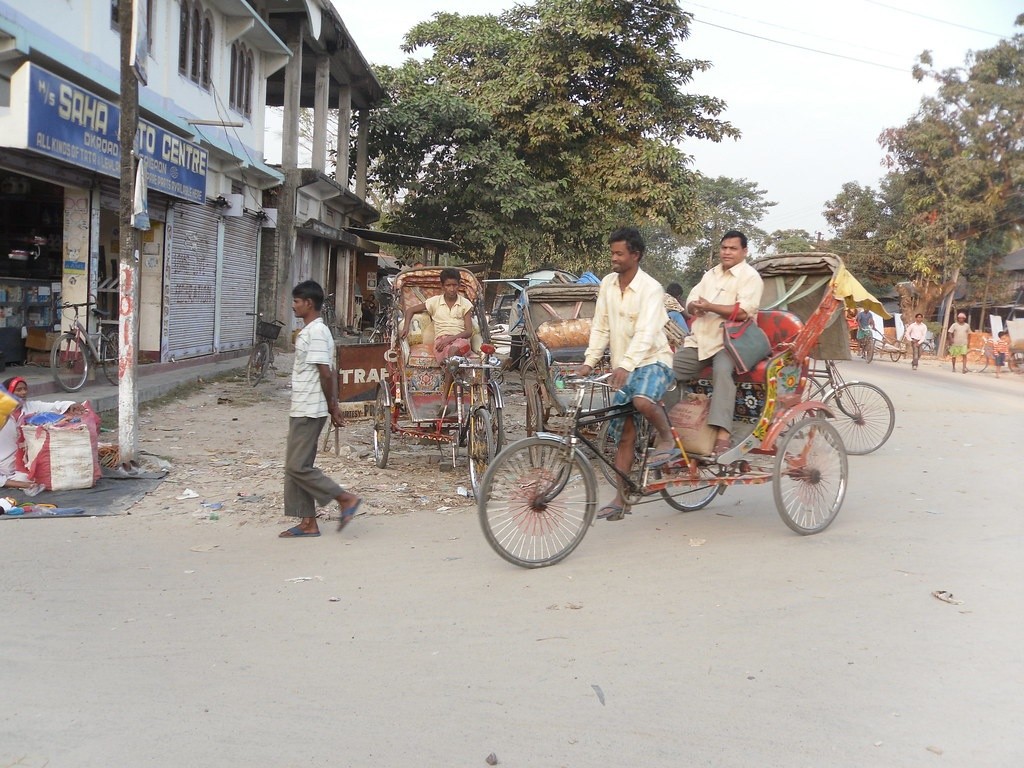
[948,312,973,374]
[672,232,764,457]
[279,281,361,537]
[993,331,1009,379]
[0,377,85,487]
[857,306,876,357]
[906,313,928,370]
[362,294,376,331]
[666,282,690,353]
[400,268,475,417]
[575,226,675,519]
[844,306,859,341]
[509,288,525,372]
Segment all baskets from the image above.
[257,321,282,339]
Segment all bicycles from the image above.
[49,293,119,393]
[965,336,1023,372]
[244,312,286,388]
[491,320,541,397]
[921,338,937,354]
[777,355,897,455]
[357,296,394,344]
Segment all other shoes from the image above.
[436,405,450,416]
[963,368,970,374]
[912,364,917,370]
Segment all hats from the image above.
[958,313,966,318]
[2,376,27,393]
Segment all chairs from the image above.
[610,391,614,400]
[405,286,478,395]
[535,315,609,402]
[686,307,808,421]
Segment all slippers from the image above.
[279,527,321,538]
[338,498,363,530]
[710,437,734,457]
[645,447,681,466]
[595,506,633,518]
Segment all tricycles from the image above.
[475,248,893,570]
[516,280,653,491]
[378,265,503,506]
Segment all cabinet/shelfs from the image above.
[0,276,64,367]
[0,195,63,282]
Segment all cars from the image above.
[492,291,515,324]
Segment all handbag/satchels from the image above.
[722,304,773,374]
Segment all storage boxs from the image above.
[25,328,61,352]
[652,392,719,456]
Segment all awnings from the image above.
[342,225,461,266]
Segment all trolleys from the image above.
[857,325,908,364]
